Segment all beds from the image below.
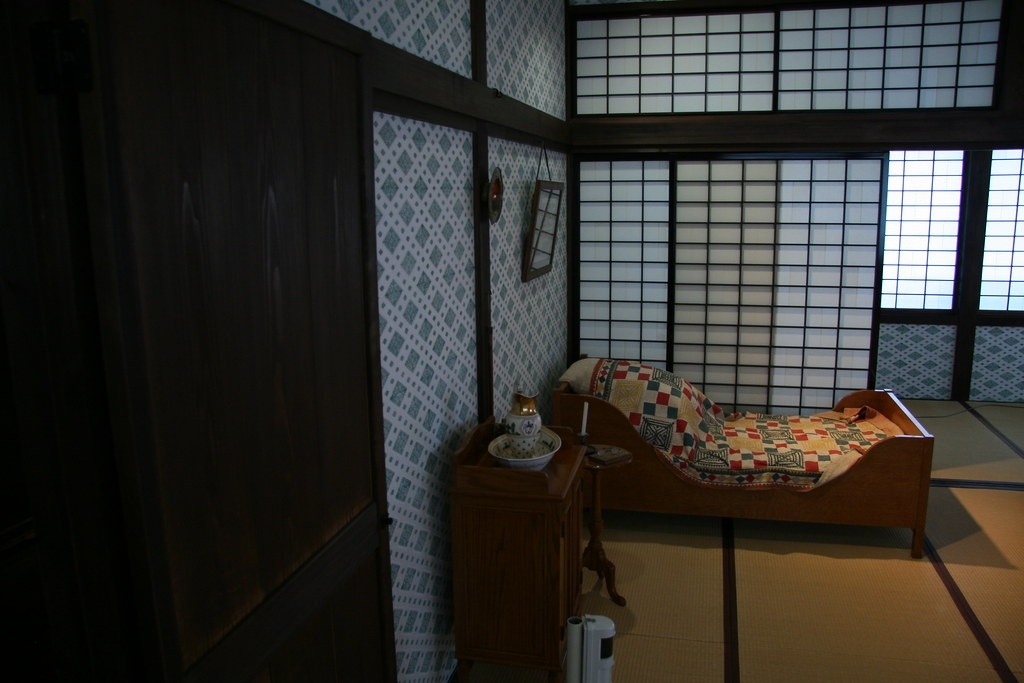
[552,354,935,559]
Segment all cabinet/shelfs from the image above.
[448,424,586,683]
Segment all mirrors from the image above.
[521,180,565,283]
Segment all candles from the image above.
[582,401,589,435]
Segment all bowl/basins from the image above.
[488,427,561,472]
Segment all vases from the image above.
[506,392,542,458]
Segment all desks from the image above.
[583,444,634,607]
